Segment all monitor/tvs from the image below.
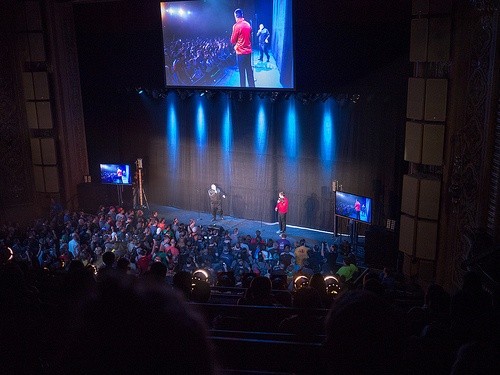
[98,162,132,185]
[333,190,373,224]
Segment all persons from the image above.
[207,184,226,221]
[274,192,289,237]
[100,167,126,183]
[164,33,236,86]
[354,199,360,220]
[256,24,271,62]
[230,8,255,87]
[0,205,500,375]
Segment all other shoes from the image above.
[211,218,216,222]
[220,217,224,220]
[276,230,282,234]
[279,232,286,237]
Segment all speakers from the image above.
[365,229,399,269]
[77,183,98,211]
[207,224,224,233]
